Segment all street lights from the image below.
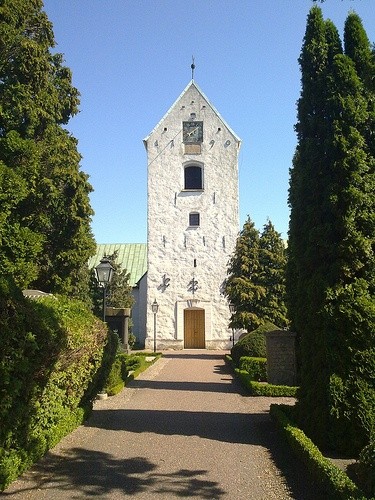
[228,301,236,346]
[94,252,115,323]
[151,299,159,353]
